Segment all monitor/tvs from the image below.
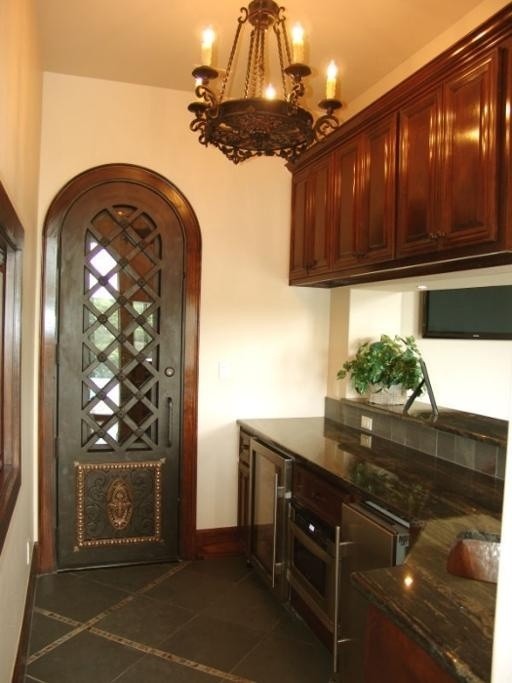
[422,285,512,341]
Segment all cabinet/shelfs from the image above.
[289,460,340,669]
[285,133,330,288]
[330,111,398,287]
[338,496,412,681]
[399,39,512,277]
[237,419,292,597]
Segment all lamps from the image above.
[187,1,343,165]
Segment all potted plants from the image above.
[339,335,420,406]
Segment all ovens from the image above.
[287,508,333,635]
